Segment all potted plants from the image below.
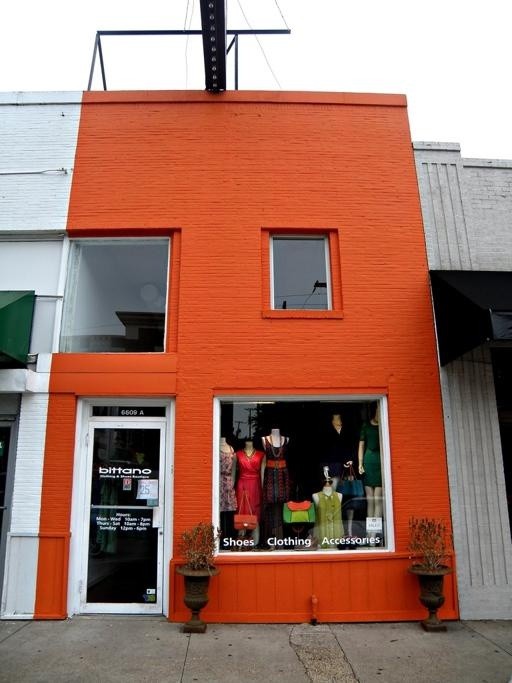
[406,516,452,632]
[175,521,223,634]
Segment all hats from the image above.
[369,408,379,426]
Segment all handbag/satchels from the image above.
[234,514,257,530]
[282,501,317,523]
[338,465,364,496]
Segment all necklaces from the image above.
[243,448,256,458]
[373,417,378,423]
[334,421,341,427]
[270,435,282,456]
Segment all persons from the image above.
[322,412,358,549]
[219,435,238,549]
[231,441,267,548]
[311,480,344,549]
[358,407,384,546]
[259,428,290,552]
